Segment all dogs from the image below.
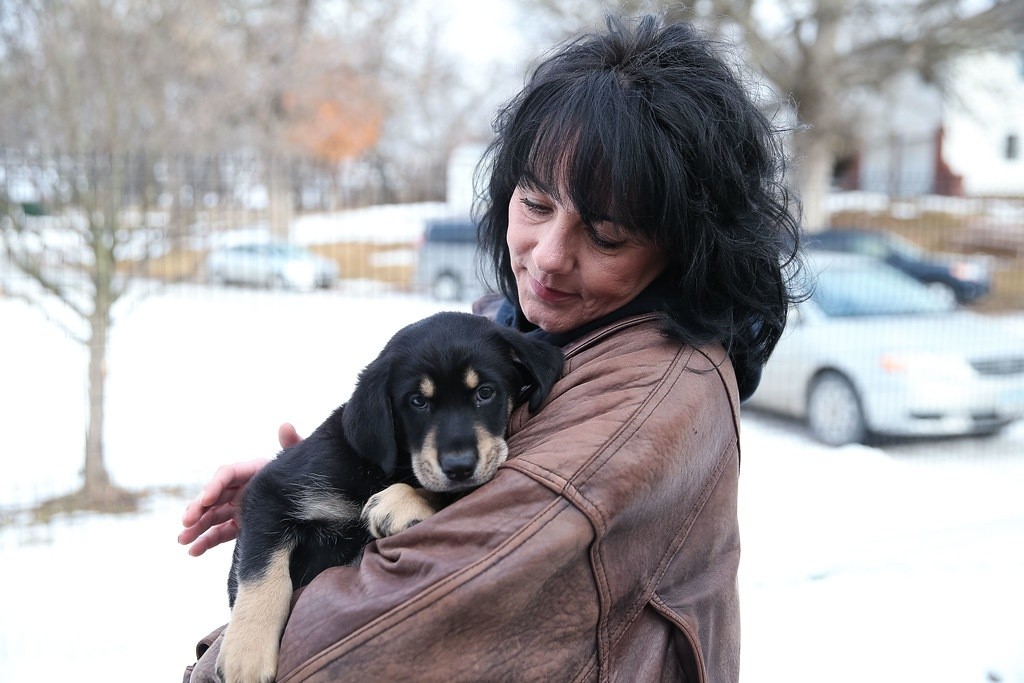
[215,312,563,683]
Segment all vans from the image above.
[415,216,507,301]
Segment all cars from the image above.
[744,252,1024,447]
[778,227,987,301]
[204,230,336,289]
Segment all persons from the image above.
[179,5,821,683]
